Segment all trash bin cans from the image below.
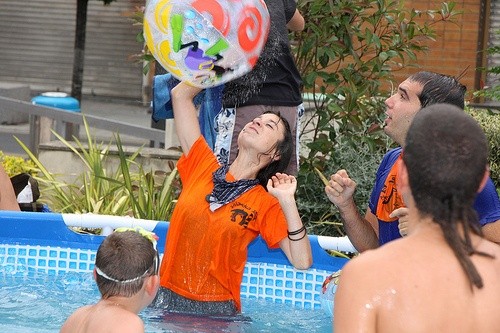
[30,90,82,164]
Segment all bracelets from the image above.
[286,226,307,241]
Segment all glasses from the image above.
[114,227,158,252]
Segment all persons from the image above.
[0,162,21,211]
[60,230,159,333]
[212,0,305,177]
[333,102,500,332]
[146,79,314,316]
[326,69,500,253]
